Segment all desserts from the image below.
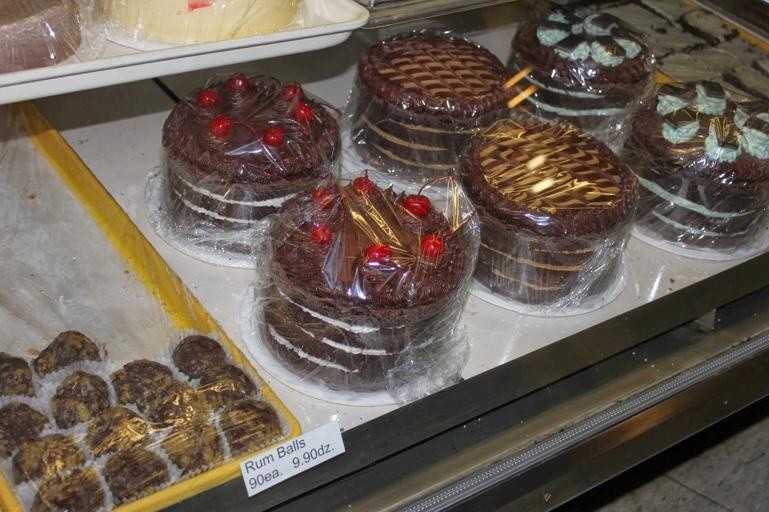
[1,330,283,511]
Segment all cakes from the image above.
[2,1,82,73]
[84,2,300,45]
[161,74,339,254]
[506,6,655,143]
[619,80,768,260]
[257,168,478,393]
[350,30,506,188]
[459,114,638,305]
